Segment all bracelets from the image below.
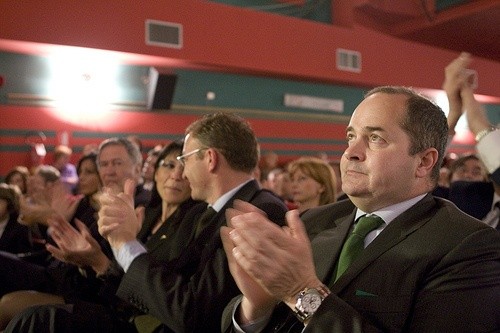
[473,123,497,143]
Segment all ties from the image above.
[331,212,385,288]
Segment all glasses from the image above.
[177,147,216,165]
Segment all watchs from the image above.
[292,285,331,323]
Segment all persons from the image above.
[221,86,500,333]
[96,112,291,333]
[432,150,499,231]
[44,140,209,333]
[133,143,164,209]
[438,52,500,233]
[255,150,349,210]
[58,137,154,290]
[1,129,99,333]
